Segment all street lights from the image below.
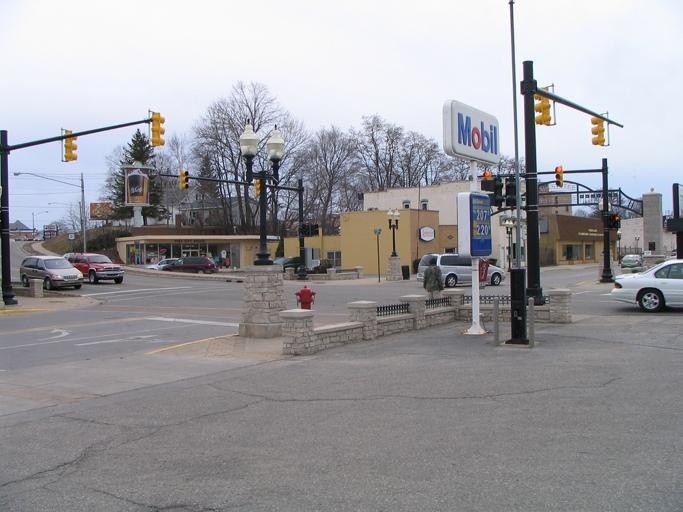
[238,117,286,265]
[386,206,399,257]
[12,171,87,253]
[31,210,48,240]
[48,201,82,231]
[616,231,621,266]
[634,235,640,254]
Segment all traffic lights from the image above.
[555,166,563,187]
[152,112,165,146]
[590,115,604,146]
[65,130,78,161]
[609,214,620,227]
[180,168,189,193]
[534,87,551,126]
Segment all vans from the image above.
[19,255,84,289]
[416,253,505,287]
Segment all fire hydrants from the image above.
[293,285,314,309]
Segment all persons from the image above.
[422,257,445,299]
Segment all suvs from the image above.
[163,256,216,275]
[67,253,124,285]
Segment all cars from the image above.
[145,258,183,272]
[297,259,331,274]
[620,254,641,267]
[273,257,300,272]
[32,236,43,241]
[15,236,28,241]
[608,259,683,312]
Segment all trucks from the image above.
[42,223,59,240]
